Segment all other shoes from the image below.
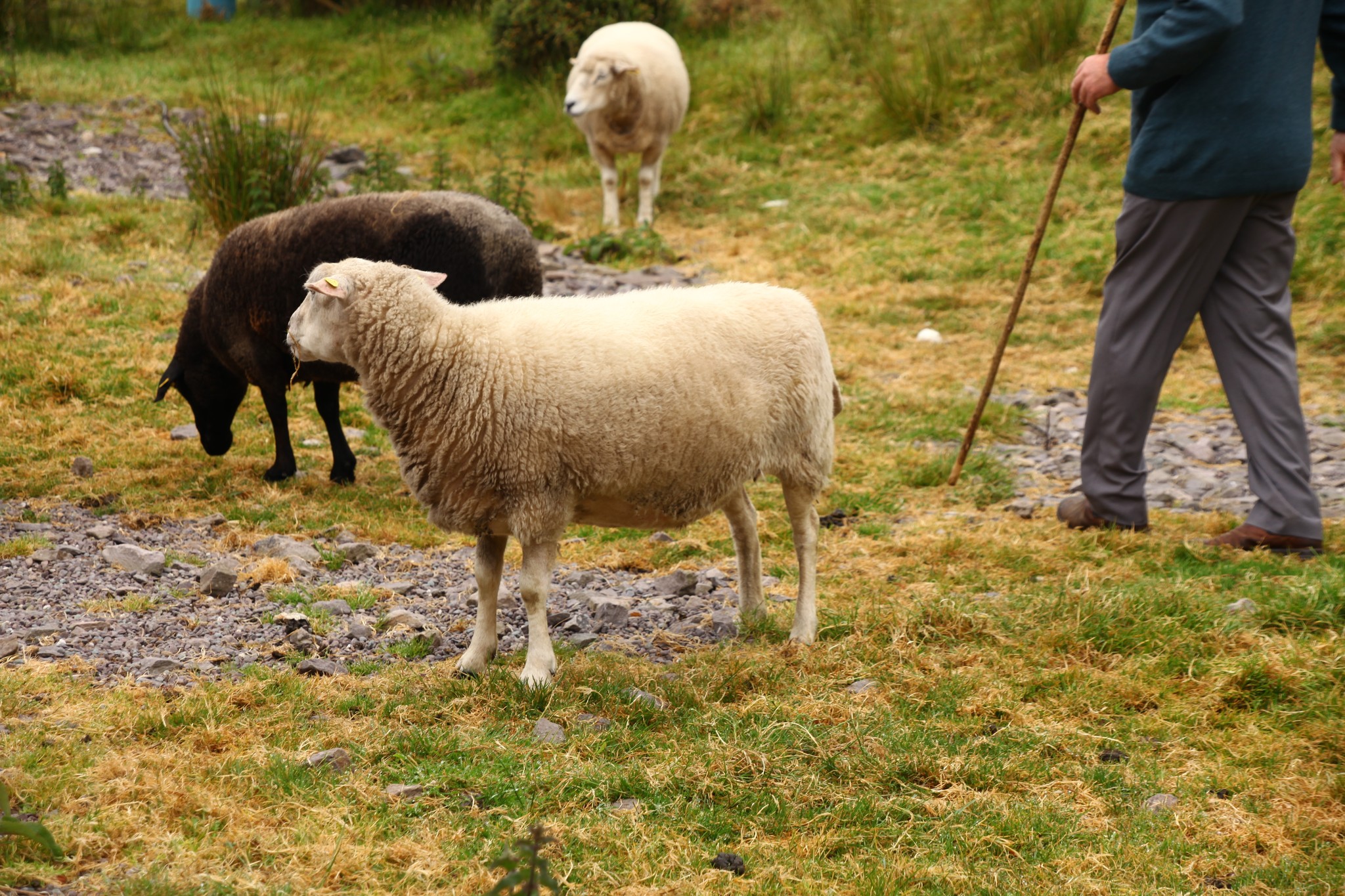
[1057,493,1152,536]
[1206,521,1326,557]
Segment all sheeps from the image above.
[560,18,695,236]
[283,253,847,692]
[148,186,544,487]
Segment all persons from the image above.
[1057,0,1345,550]
